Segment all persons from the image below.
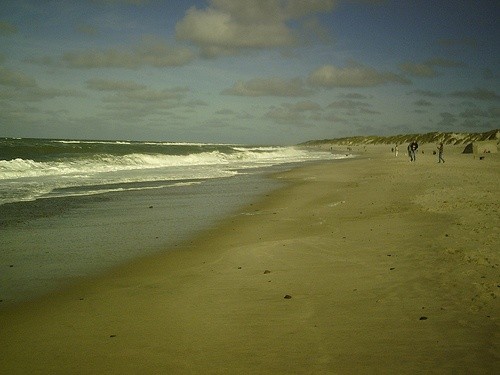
[408,142,413,162]
[478,150,491,161]
[411,139,418,162]
[391,148,394,152]
[436,142,445,164]
[395,144,399,157]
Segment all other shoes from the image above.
[439,160,441,163]
[443,161,445,163]
[410,158,416,161]
